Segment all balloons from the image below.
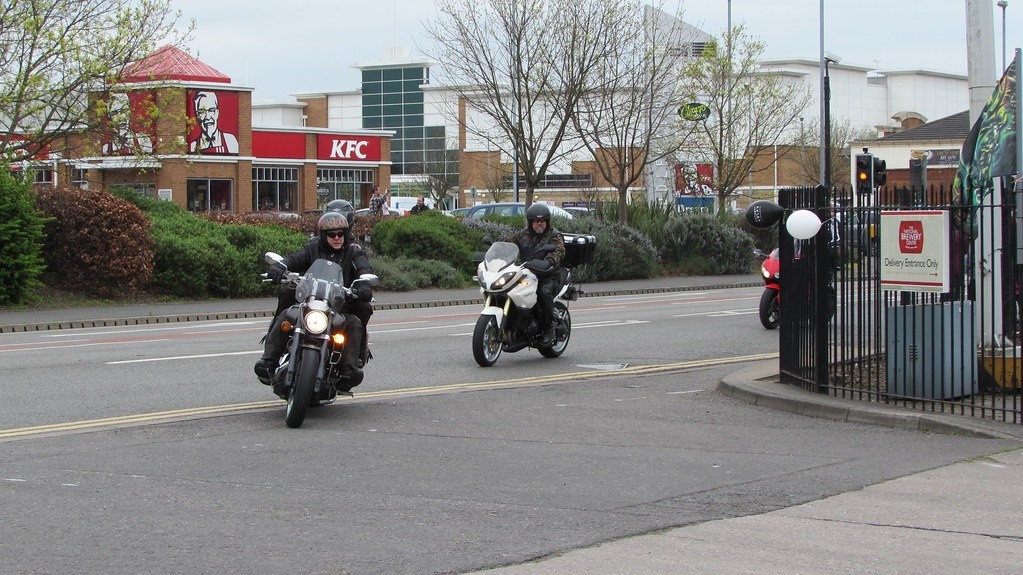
[745,200,784,228]
[786,209,823,240]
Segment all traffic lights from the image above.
[873,157,887,188]
[855,154,873,193]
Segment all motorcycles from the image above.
[754,246,836,330]
[471,234,595,369]
[260,251,381,428]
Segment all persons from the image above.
[509,204,566,345]
[939,229,969,302]
[255,199,374,392]
[828,204,839,245]
[410,196,429,215]
[369,185,390,216]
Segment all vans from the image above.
[468,202,576,223]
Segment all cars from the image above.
[238,205,412,223]
[842,210,881,258]
[561,207,588,218]
[450,207,485,218]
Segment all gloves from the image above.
[352,280,372,304]
[267,262,287,286]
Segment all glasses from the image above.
[325,229,344,237]
[533,218,545,223]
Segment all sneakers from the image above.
[538,321,555,344]
[254,357,273,385]
[336,368,364,392]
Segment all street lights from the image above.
[997,0,1008,75]
[822,51,843,198]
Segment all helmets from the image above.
[526,203,550,219]
[323,199,355,228]
[317,212,351,232]
